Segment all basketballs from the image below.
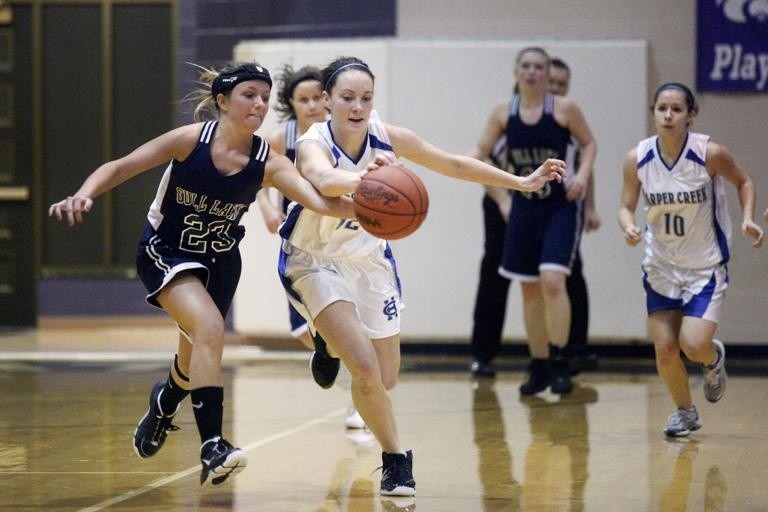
[353,165,428,240]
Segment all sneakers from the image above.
[560,353,583,374]
[307,327,339,389]
[519,363,550,395]
[702,339,728,402]
[132,378,181,461]
[471,360,493,378]
[662,405,702,437]
[550,360,571,393]
[380,450,415,496]
[344,410,367,428]
[200,438,247,491]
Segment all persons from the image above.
[275,55,567,498]
[620,81,767,440]
[257,64,372,429]
[49,60,359,490]
[475,43,597,402]
[465,57,601,380]
